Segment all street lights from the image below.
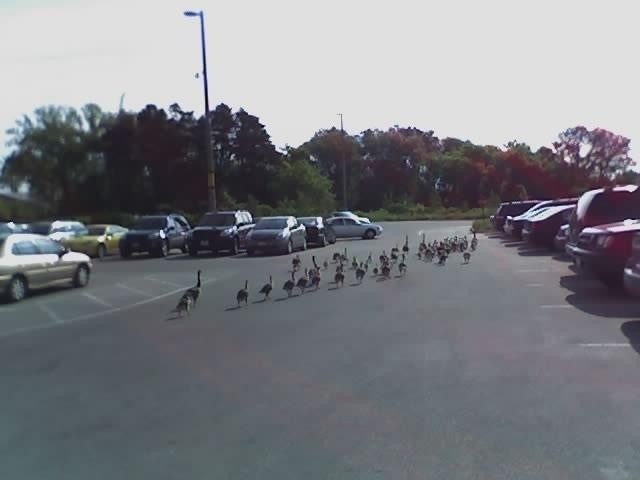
[183,8,218,214]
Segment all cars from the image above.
[489,183,640,304]
[243,215,309,257]
[330,211,370,225]
[63,223,129,259]
[296,216,335,248]
[325,216,384,240]
[0,219,87,242]
[0,233,94,303]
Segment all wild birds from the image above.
[282,248,348,298]
[182,270,204,307]
[258,275,272,300]
[403,236,410,249]
[352,245,407,284]
[418,231,477,267]
[170,295,191,318]
[237,280,249,305]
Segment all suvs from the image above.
[183,209,256,256]
[118,214,191,259]
